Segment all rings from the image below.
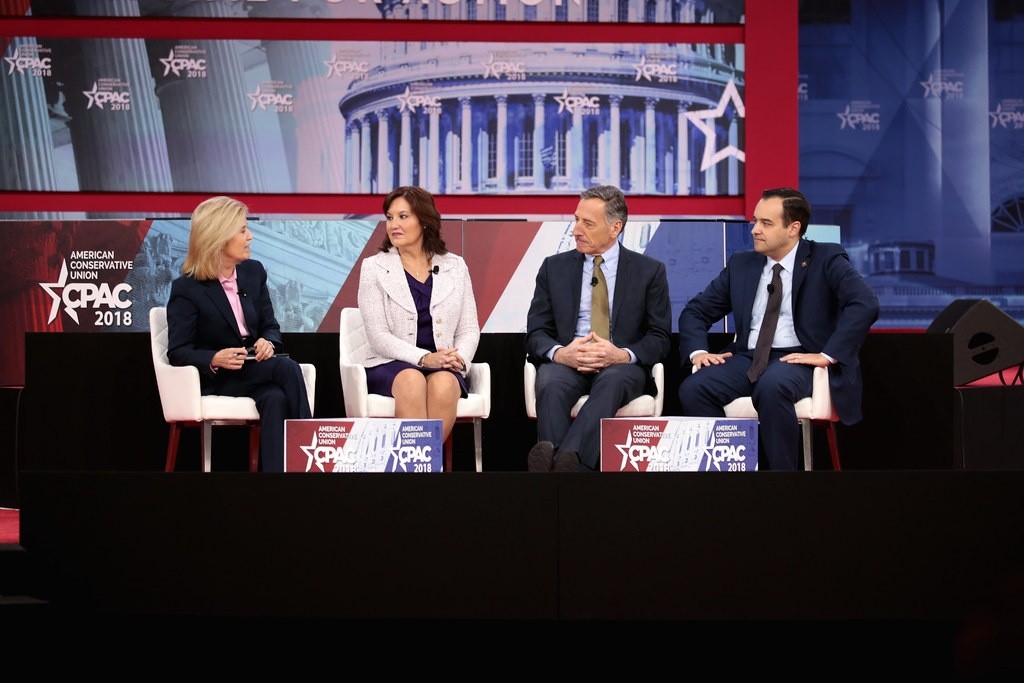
[799,355,801,358]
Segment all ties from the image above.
[591,256,609,343]
[746,264,784,383]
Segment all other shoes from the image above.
[528,441,554,472]
[557,449,580,472]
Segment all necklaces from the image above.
[413,269,427,276]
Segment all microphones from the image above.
[592,277,598,286]
[767,284,774,295]
[238,288,247,298]
[433,266,439,274]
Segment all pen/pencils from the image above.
[232,346,257,357]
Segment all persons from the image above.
[166,196,313,474]
[358,186,480,444]
[679,187,879,470]
[525,185,673,472]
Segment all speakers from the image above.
[924,298,1024,387]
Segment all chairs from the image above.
[523,349,665,422]
[148,306,318,474]
[690,360,832,473]
[339,307,492,473]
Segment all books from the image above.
[245,353,289,360]
